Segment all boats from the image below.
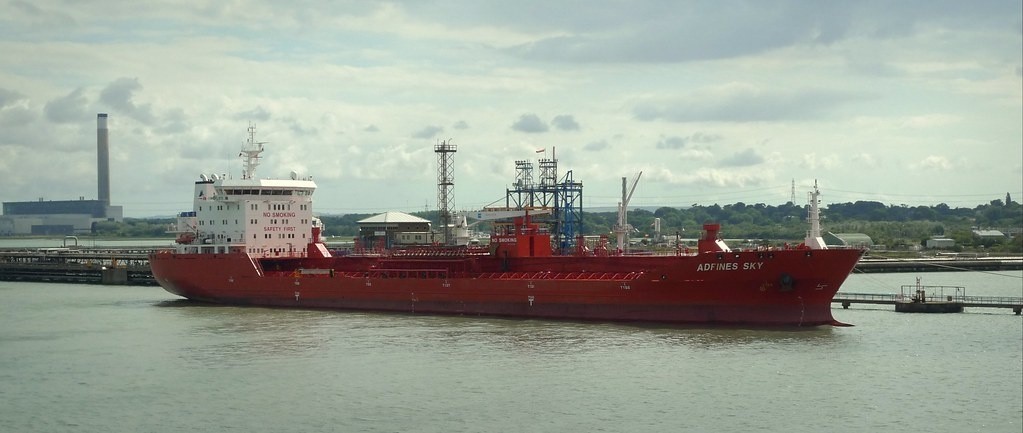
[148,120,871,329]
